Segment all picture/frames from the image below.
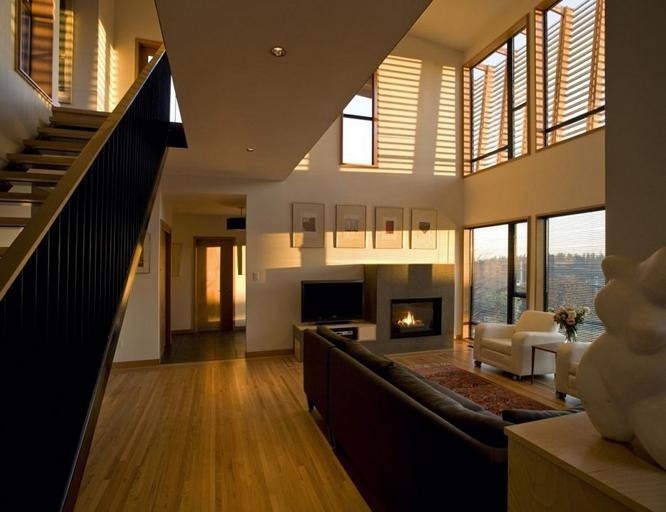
[55,7,74,106]
[288,201,326,249]
[407,206,440,250]
[373,205,404,250]
[132,233,151,274]
[332,203,367,249]
[12,0,56,105]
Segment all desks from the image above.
[530,341,582,396]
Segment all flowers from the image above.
[549,301,593,341]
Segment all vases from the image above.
[563,327,577,344]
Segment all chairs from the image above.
[553,338,597,402]
[469,308,566,383]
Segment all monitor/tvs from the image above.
[301,279,364,325]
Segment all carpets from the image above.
[407,356,561,416]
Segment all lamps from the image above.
[223,205,246,232]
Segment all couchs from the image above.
[298,320,585,510]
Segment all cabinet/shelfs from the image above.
[501,407,666,512]
[291,318,378,363]
